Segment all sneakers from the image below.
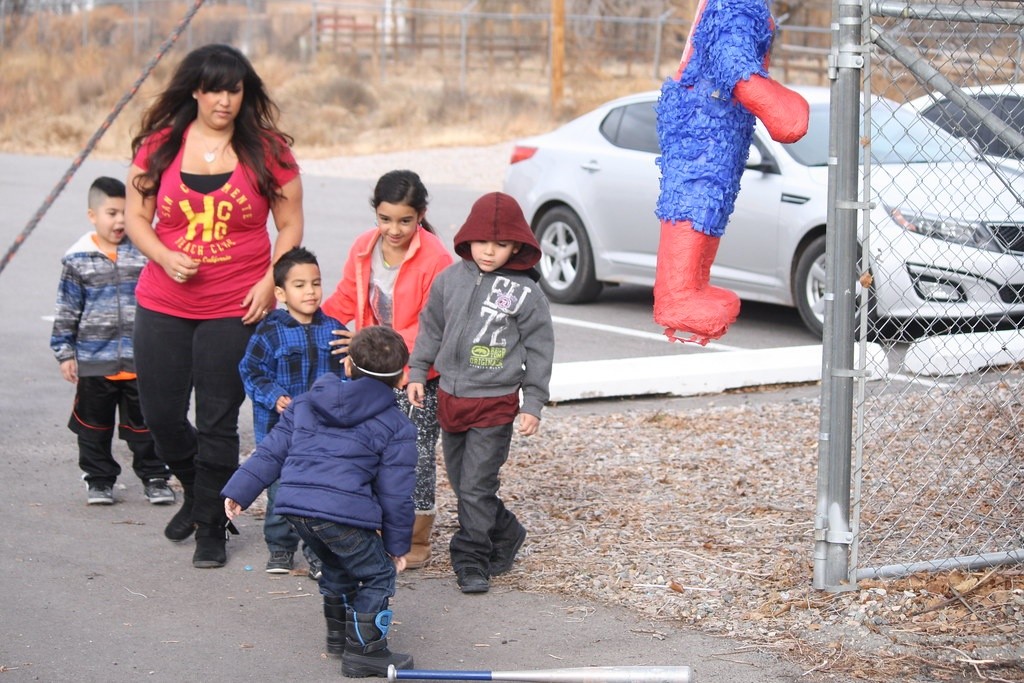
[489,515,527,576]
[144,479,174,503]
[88,484,113,505]
[458,567,490,593]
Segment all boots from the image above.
[324,591,363,659]
[404,510,436,569]
[154,447,196,542]
[193,456,237,568]
[342,611,413,678]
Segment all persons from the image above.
[221,326,413,678]
[50,177,174,505]
[406,193,555,594]
[319,170,453,566]
[123,43,303,568]
[238,248,349,581]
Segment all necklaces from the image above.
[196,121,223,162]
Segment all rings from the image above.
[176,273,181,278]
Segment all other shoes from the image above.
[266,551,293,573]
[308,567,322,579]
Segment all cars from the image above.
[505,86,1024,341]
[903,84,1024,173]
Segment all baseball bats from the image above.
[387,664,690,683]
